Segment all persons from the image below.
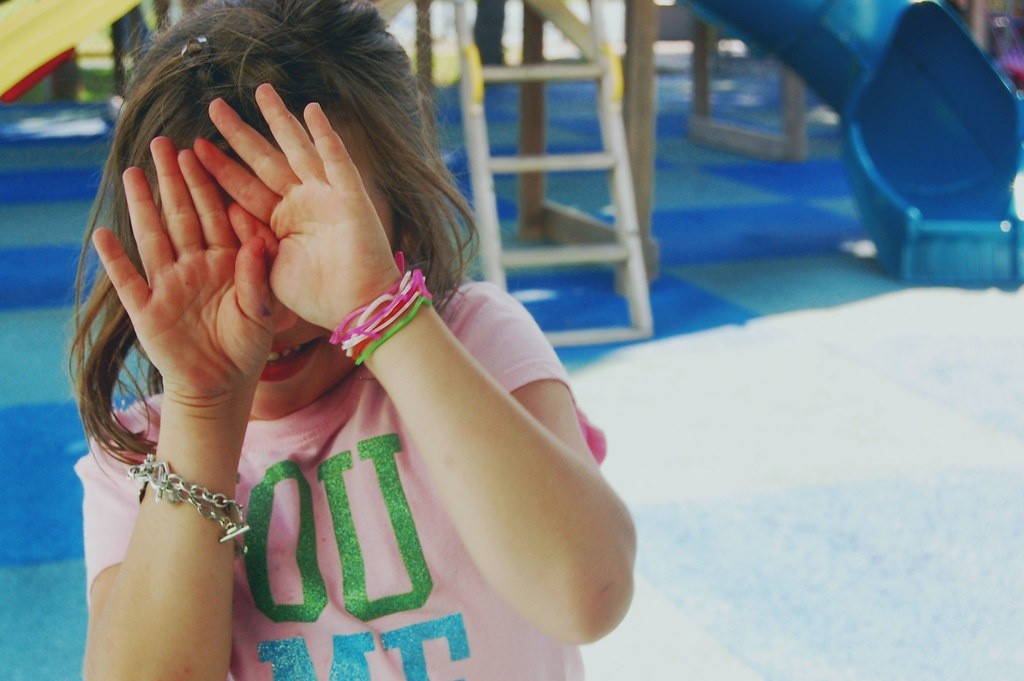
[68,0,637,681]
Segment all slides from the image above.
[673,0,1024,292]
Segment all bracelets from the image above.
[329,251,432,365]
[126,453,250,561]
